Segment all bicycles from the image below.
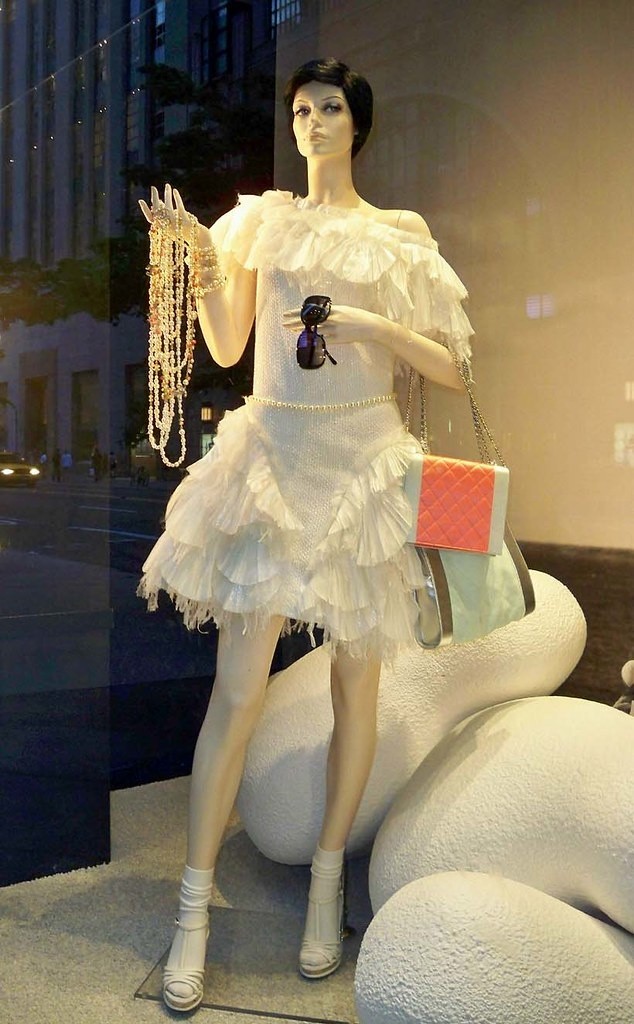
[129,466,149,489]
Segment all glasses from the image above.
[296,295,337,370]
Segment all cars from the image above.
[0,451,42,488]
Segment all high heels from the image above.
[298,871,344,980]
[161,913,209,1011]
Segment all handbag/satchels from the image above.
[397,337,509,557]
[414,339,537,648]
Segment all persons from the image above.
[136,465,149,488]
[28,447,73,483]
[109,451,118,479]
[92,448,103,482]
[133,58,473,1014]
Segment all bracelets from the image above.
[184,243,228,296]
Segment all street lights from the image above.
[0,397,19,450]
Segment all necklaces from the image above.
[145,198,199,469]
